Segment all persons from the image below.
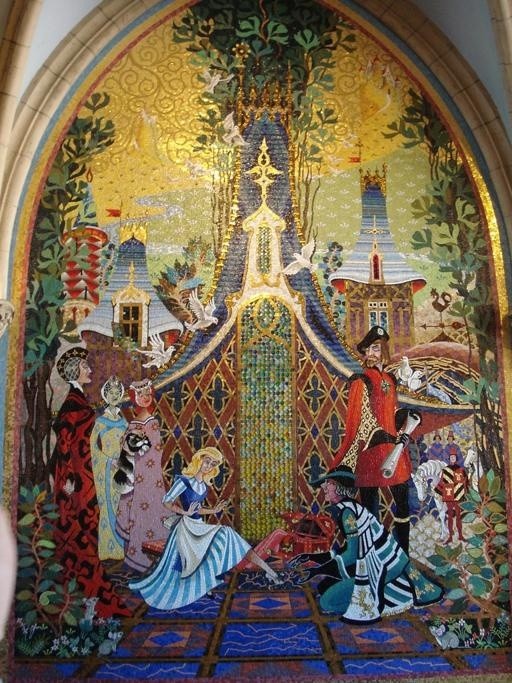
[53,343,132,619]
[86,373,130,563]
[271,460,447,623]
[323,324,424,559]
[117,375,174,570]
[129,445,287,610]
[428,444,474,543]
[442,431,464,465]
[426,433,445,461]
